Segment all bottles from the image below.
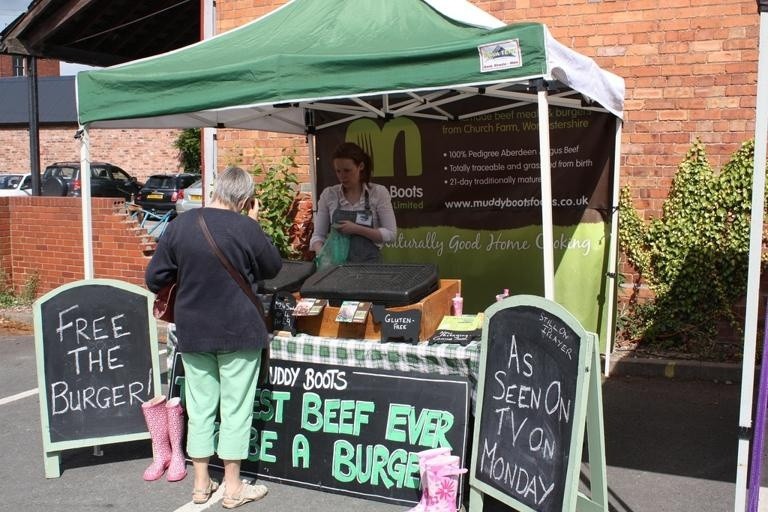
[450,292,464,316]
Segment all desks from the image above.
[161,320,480,428]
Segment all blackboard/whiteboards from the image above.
[31,279,161,453]
[469,294,594,512]
[167,351,471,511]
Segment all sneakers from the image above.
[221,483,270,509]
[191,478,221,504]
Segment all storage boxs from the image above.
[254,256,313,295]
[299,259,441,310]
[291,277,463,343]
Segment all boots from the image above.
[401,446,451,512]
[141,395,173,482]
[166,398,188,481]
[427,455,468,512]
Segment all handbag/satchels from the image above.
[258,340,271,387]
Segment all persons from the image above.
[99,170,107,176]
[309,143,398,261]
[144,167,282,510]
[130,177,142,196]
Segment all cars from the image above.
[1,160,204,215]
